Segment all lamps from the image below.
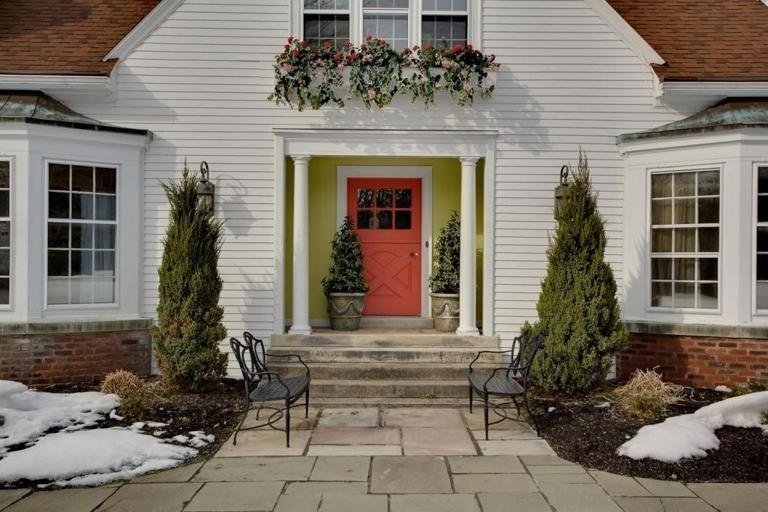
[195,161,213,218]
[554,165,568,220]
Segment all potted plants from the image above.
[320,215,369,330]
[429,209,461,332]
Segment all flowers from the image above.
[267,36,501,112]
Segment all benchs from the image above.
[467,327,544,440]
[230,332,311,448]
